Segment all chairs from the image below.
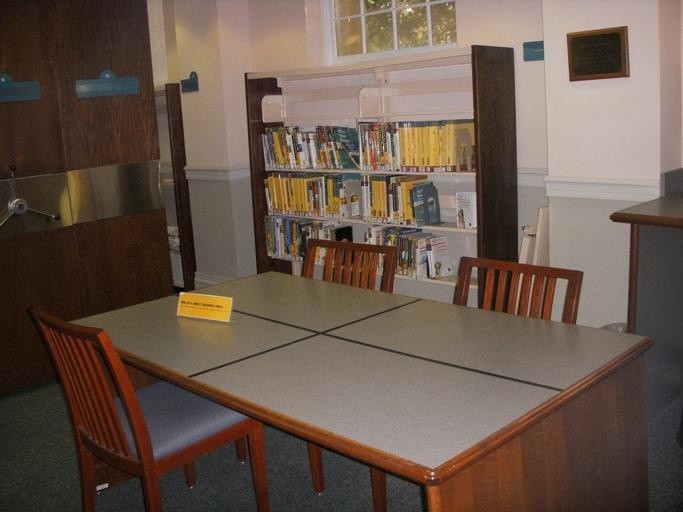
[308,255,583,512]
[300,238,398,293]
[32,308,270,511]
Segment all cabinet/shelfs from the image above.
[0,0,176,401]
[243,45,518,314]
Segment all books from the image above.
[260,120,476,280]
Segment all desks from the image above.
[69,272,653,512]
[610,194,682,338]
